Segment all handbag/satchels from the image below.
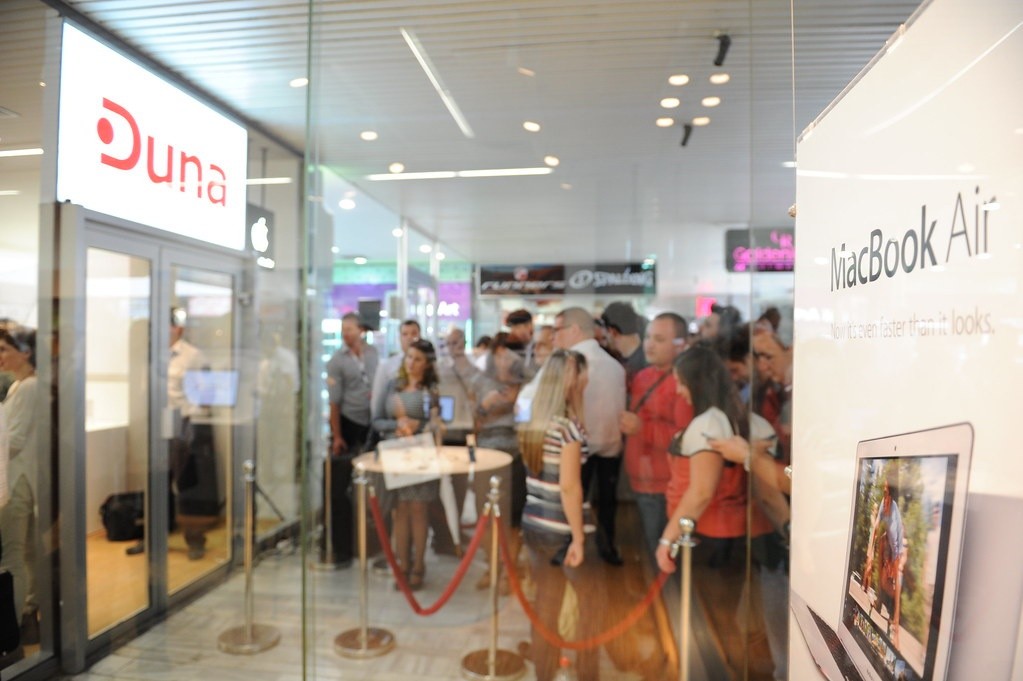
[100,486,175,541]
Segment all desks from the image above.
[352,446,513,627]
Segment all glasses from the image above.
[552,324,570,333]
[752,352,771,364]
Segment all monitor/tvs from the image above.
[182,368,243,408]
[438,393,455,424]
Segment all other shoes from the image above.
[126,541,145,554]
[598,543,625,565]
[499,564,511,596]
[480,563,501,590]
[189,543,202,559]
[432,537,463,558]
[395,571,420,590]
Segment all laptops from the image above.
[790,421,975,681]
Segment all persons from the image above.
[125,306,216,560]
[327,301,793,681]
[861,468,905,651]
[0,321,41,672]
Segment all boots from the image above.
[19,608,39,646]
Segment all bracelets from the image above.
[659,538,670,546]
